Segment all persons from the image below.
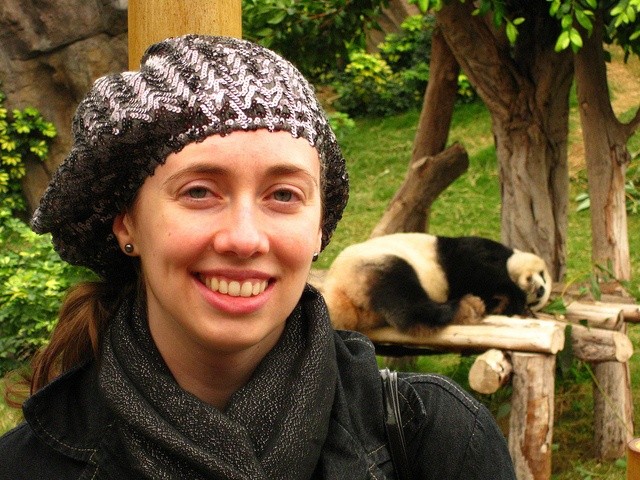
[0,32,516,480]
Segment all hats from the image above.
[30,35,349,283]
